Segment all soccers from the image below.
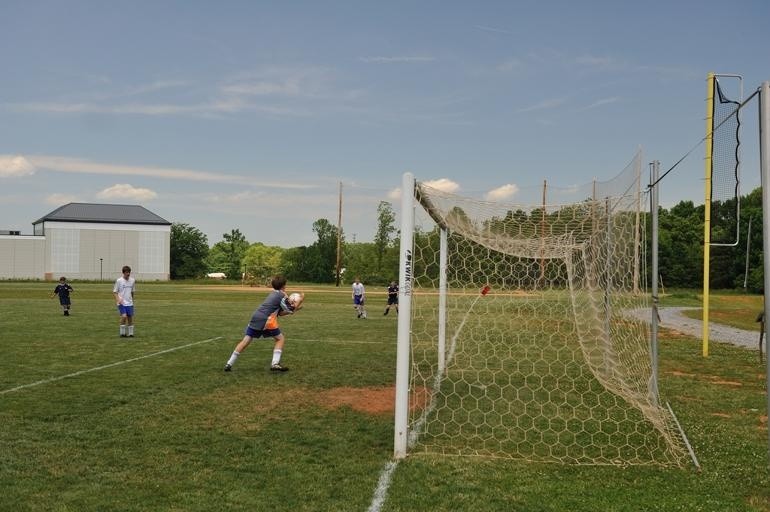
[289,292,301,302]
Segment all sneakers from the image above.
[384,308,398,315]
[120,334,134,338]
[224,365,232,371]
[270,363,289,372]
[358,313,367,319]
[63,306,71,317]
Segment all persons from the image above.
[224,276,305,372]
[384,280,399,315]
[113,265,136,338]
[50,277,73,316]
[352,273,367,319]
